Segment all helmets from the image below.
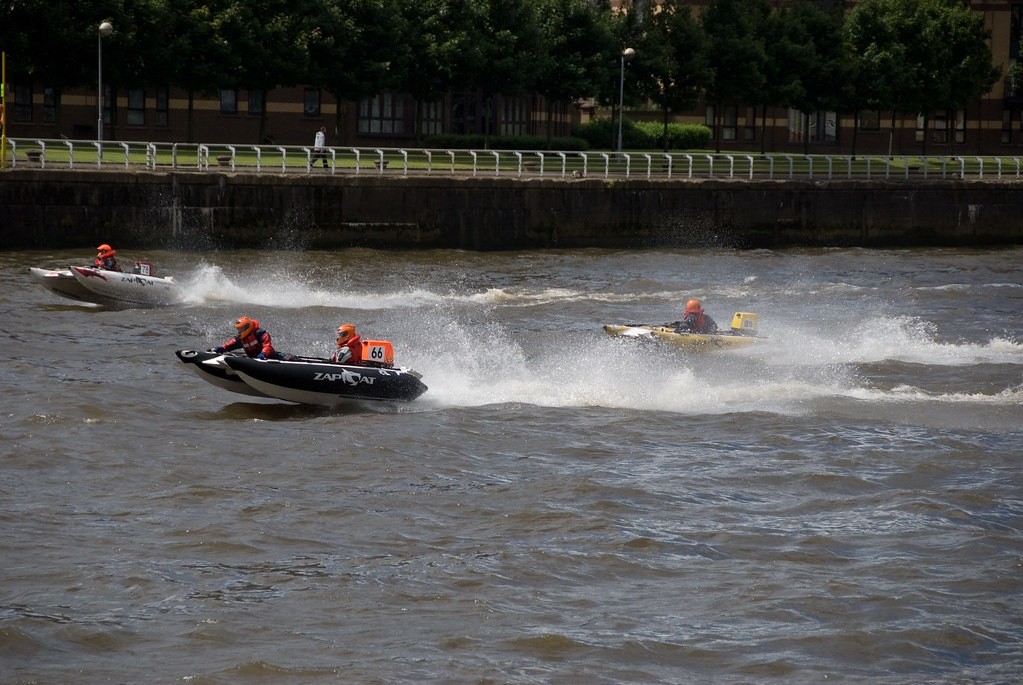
[686,300,701,314]
[337,324,355,345]
[97,244,111,258]
[235,317,255,340]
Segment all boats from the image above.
[175,340,428,407]
[603,313,770,354]
[28,261,184,308]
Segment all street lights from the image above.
[98,22,113,159]
[618,48,635,164]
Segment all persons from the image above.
[309,127,329,171]
[90,243,123,272]
[322,324,362,366]
[672,300,718,334]
[214,317,301,361]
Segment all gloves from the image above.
[213,346,225,354]
[257,352,265,360]
